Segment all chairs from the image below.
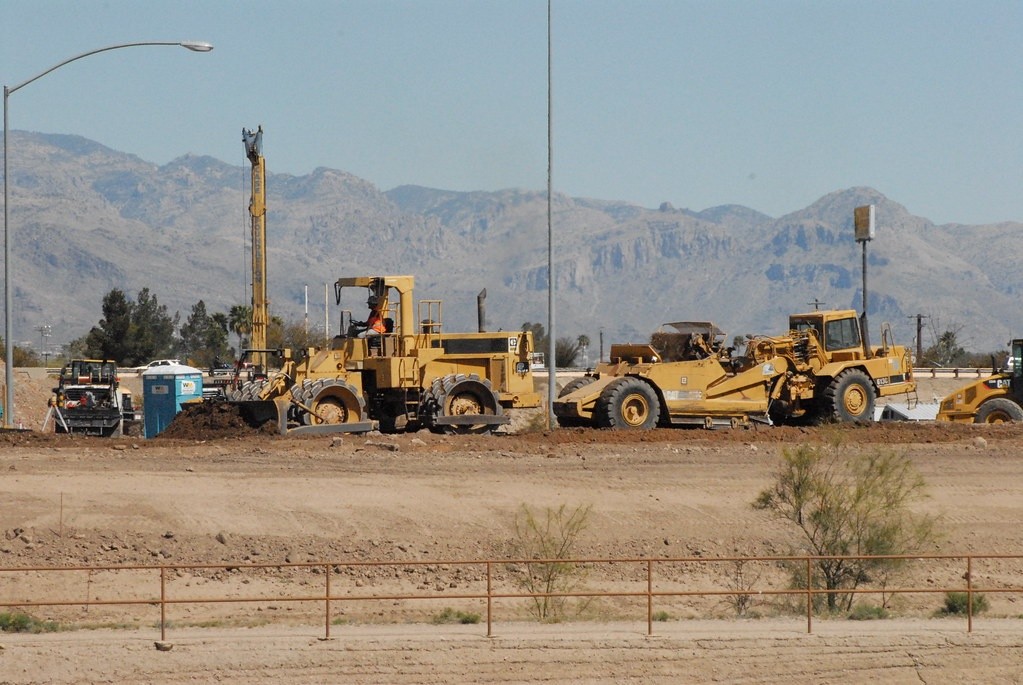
[368,318,393,347]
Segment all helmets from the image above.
[367,297,378,304]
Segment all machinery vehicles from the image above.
[46,360,140,438]
[174,124,545,436]
[930,338,1022,425]
[552,301,917,432]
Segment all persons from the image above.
[357,296,386,339]
[214,355,224,369]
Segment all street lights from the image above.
[4,40,215,429]
